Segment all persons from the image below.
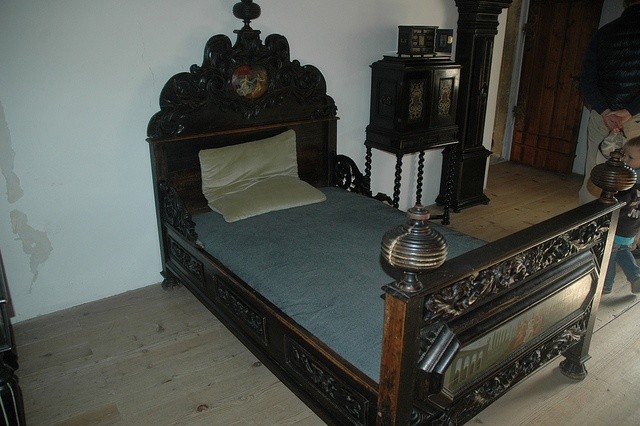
[601,136,640,294]
[578,0,640,205]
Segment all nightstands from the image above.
[364,26,464,226]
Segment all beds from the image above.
[146,0,636,426]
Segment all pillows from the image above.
[197,127,329,223]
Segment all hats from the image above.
[596,127,627,165]
